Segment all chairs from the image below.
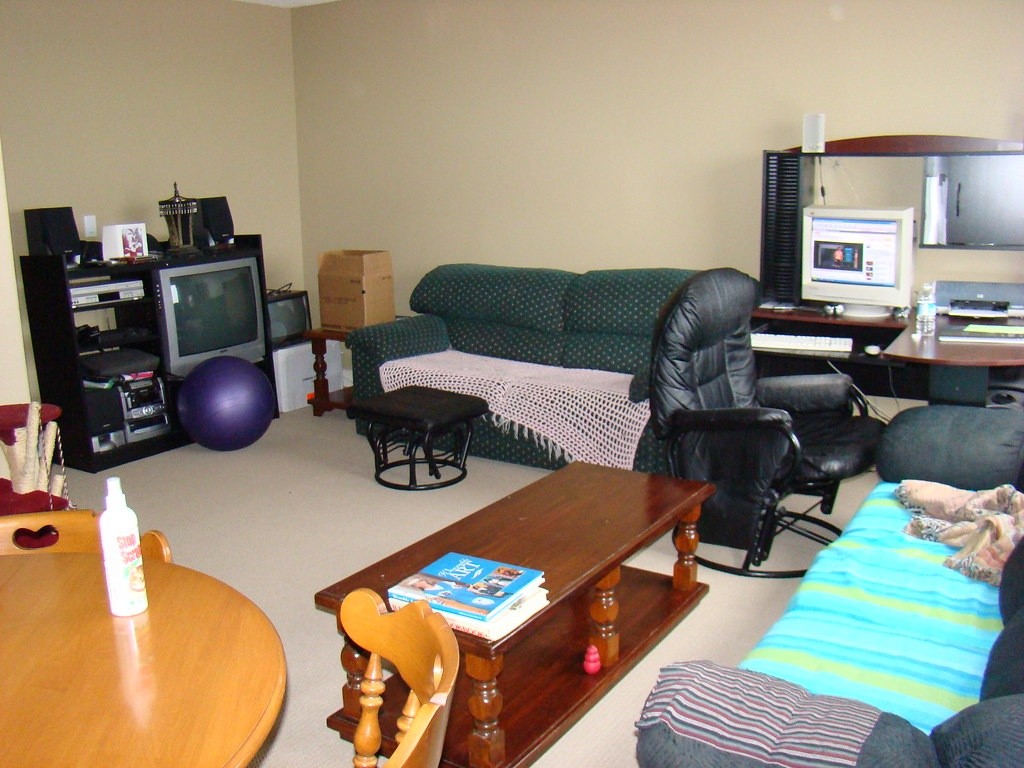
[0,509,171,561]
[651,267,886,578]
[339,589,460,768]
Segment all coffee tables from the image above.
[347,385,488,491]
[315,462,716,768]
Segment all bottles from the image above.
[916,283,937,338]
[99,476,147,617]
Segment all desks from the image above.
[749,136,1024,416]
[0,552,289,768]
[302,315,409,417]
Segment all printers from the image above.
[934,280,1024,319]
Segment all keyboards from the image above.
[750,334,853,352]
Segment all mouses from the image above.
[865,345,880,355]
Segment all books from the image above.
[948,299,1010,317]
[388,551,545,621]
[389,587,550,641]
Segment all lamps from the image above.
[158,182,201,255]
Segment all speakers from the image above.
[82,387,124,436]
[25,206,81,269]
[190,196,235,250]
[801,113,825,153]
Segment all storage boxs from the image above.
[273,340,342,412]
[317,249,394,331]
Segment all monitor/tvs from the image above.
[152,257,265,379]
[265,289,313,349]
[801,205,915,318]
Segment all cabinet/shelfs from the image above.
[19,231,274,475]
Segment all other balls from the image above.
[175,355,276,452]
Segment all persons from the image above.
[399,569,522,614]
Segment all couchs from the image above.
[634,405,1024,768]
[341,264,762,473]
